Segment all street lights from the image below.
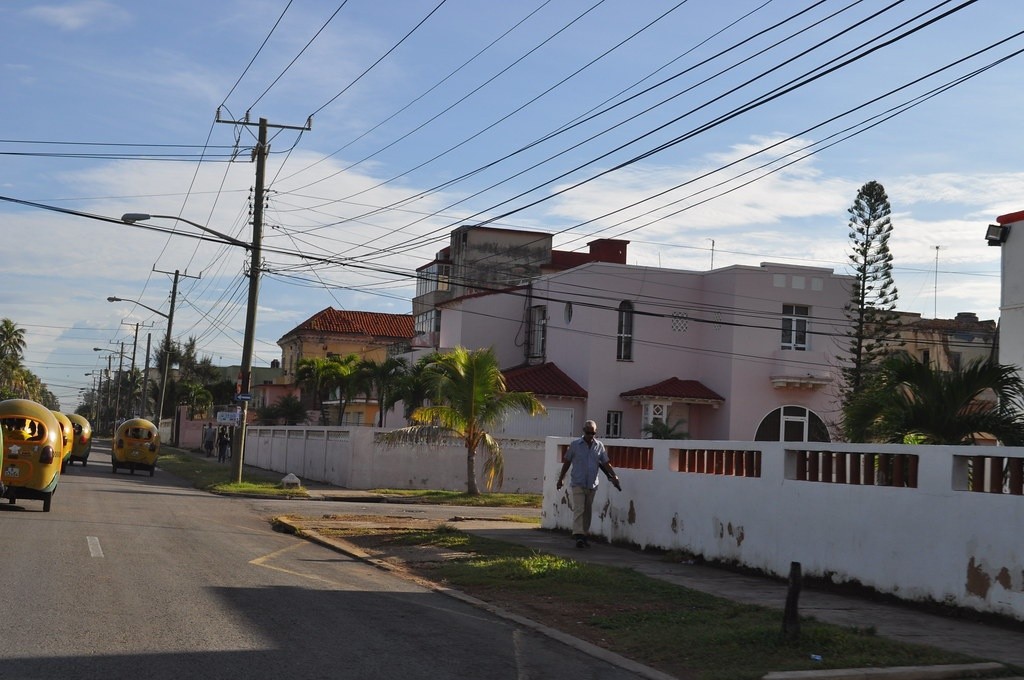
[93,347,135,420]
[78,373,111,433]
[107,297,173,429]
[121,212,263,485]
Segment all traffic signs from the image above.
[235,394,251,401]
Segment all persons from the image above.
[1,417,44,441]
[216,425,228,464]
[556,420,622,548]
[202,423,216,458]
[125,427,152,440]
[72,422,82,434]
[228,426,233,458]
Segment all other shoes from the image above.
[584,539,592,546]
[576,541,584,548]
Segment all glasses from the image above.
[585,431,596,435]
